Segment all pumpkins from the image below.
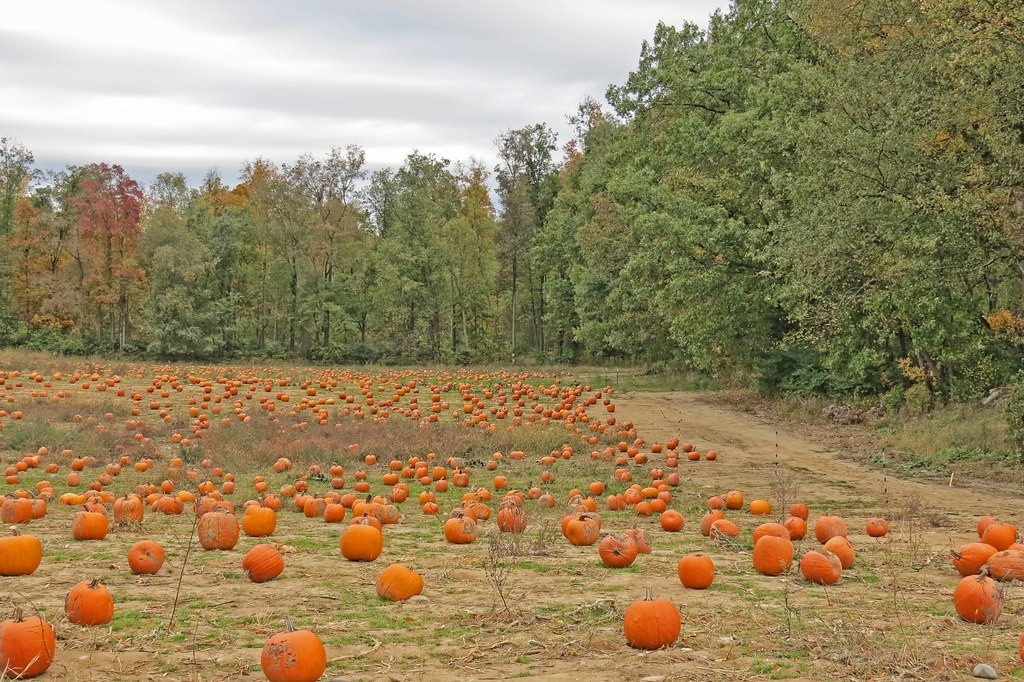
[0,364,1023,682]
